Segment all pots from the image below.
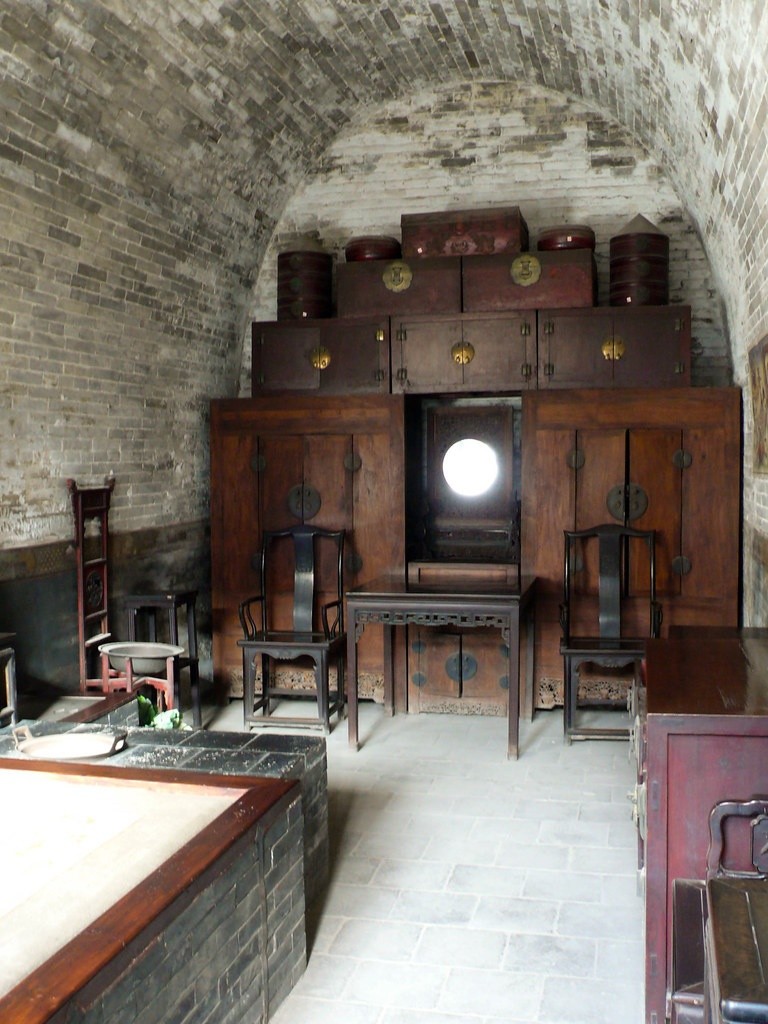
[11,724,128,759]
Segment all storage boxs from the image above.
[251,204,695,394]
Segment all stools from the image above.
[124,589,201,729]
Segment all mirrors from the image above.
[441,439,499,497]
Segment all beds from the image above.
[0,756,307,1024]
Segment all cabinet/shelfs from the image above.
[66,477,175,712]
[206,385,742,715]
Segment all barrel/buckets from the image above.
[608,234,671,305]
[277,251,333,318]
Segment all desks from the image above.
[344,571,543,762]
[627,624,768,1024]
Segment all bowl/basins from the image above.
[98,640,186,674]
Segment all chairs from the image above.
[667,793,768,1024]
[237,526,347,733]
[559,524,663,746]
